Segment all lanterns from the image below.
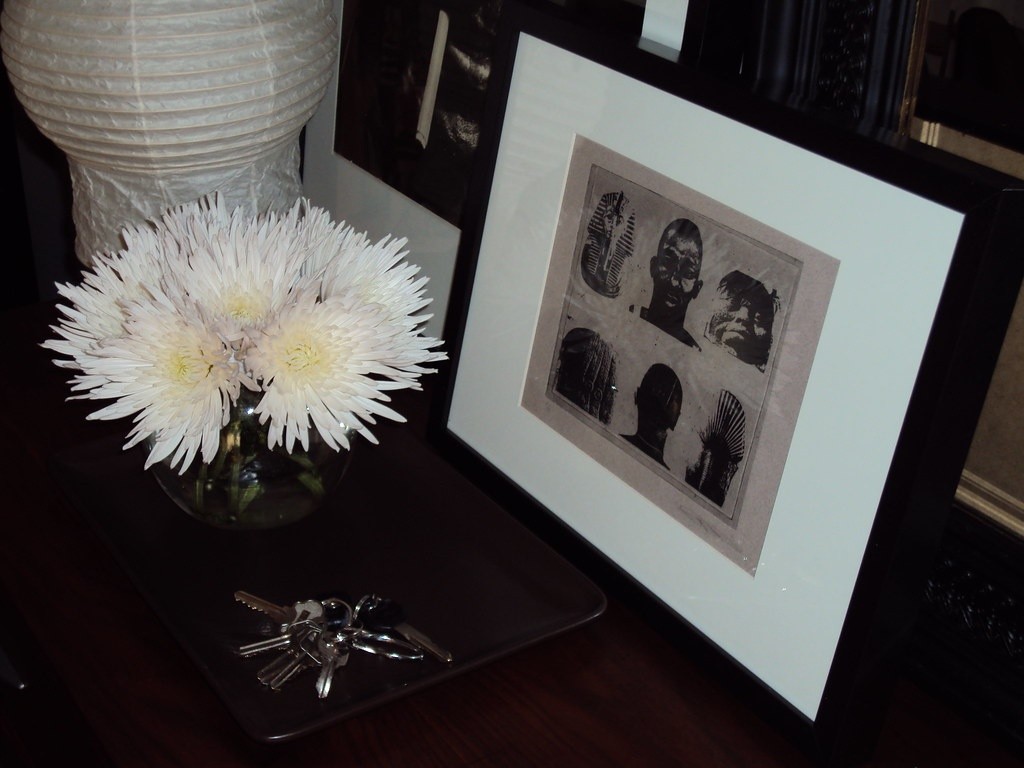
[1,1,342,267]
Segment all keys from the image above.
[235,588,451,698]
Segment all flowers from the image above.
[42,189,453,476]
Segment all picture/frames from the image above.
[420,0,1024,768]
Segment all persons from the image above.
[704,271,773,373]
[627,218,701,354]
[616,364,683,472]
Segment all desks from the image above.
[0,304,1024,768]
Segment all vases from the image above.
[140,380,359,532]
